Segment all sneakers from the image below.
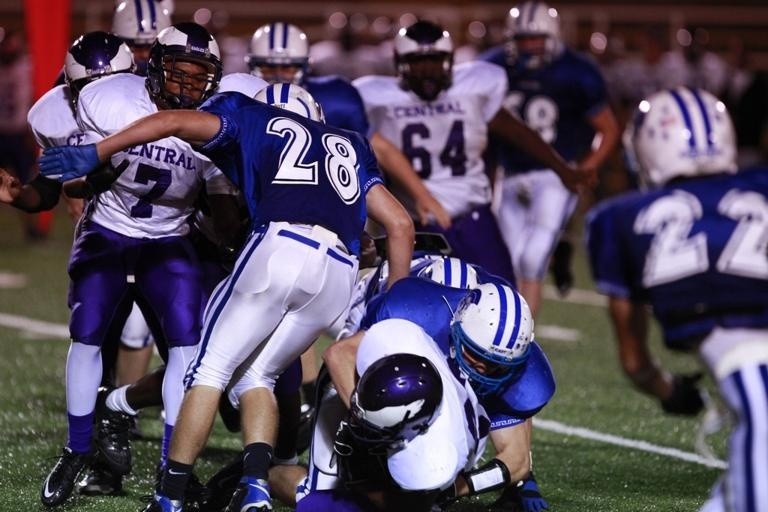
[553,241,572,295]
[40,385,272,512]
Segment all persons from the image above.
[1,1,766,512]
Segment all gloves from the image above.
[662,372,706,417]
[515,473,549,512]
[36,142,101,183]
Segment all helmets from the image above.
[331,280,534,458]
[622,86,739,194]
